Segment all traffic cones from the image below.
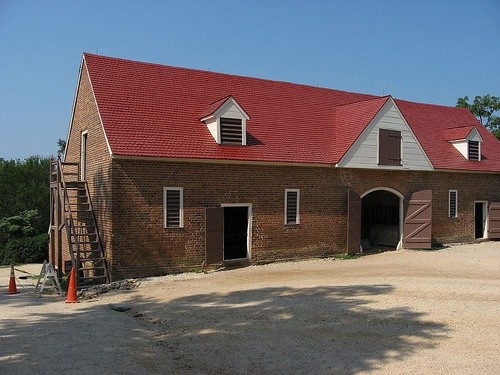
[65,266,81,303]
[5,264,21,295]
